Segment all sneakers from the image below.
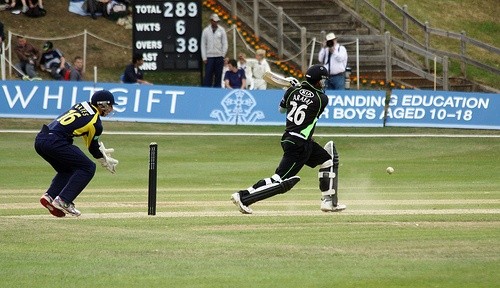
[231,192,253,214]
[52,196,81,217]
[40,193,66,219]
[320,202,348,212]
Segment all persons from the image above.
[86,0,110,19]
[12,35,39,80]
[10,0,44,13]
[222,48,272,92]
[318,31,348,91]
[0,22,6,53]
[33,88,119,219]
[39,40,74,81]
[64,55,86,81]
[123,50,153,85]
[228,65,348,215]
[199,13,229,87]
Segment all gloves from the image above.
[282,77,301,91]
[97,153,118,174]
[99,142,114,157]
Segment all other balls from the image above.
[387,167,394,174]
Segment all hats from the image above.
[305,65,328,79]
[325,33,336,41]
[210,14,221,21]
[91,89,118,105]
[42,41,53,51]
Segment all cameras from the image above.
[327,40,334,47]
